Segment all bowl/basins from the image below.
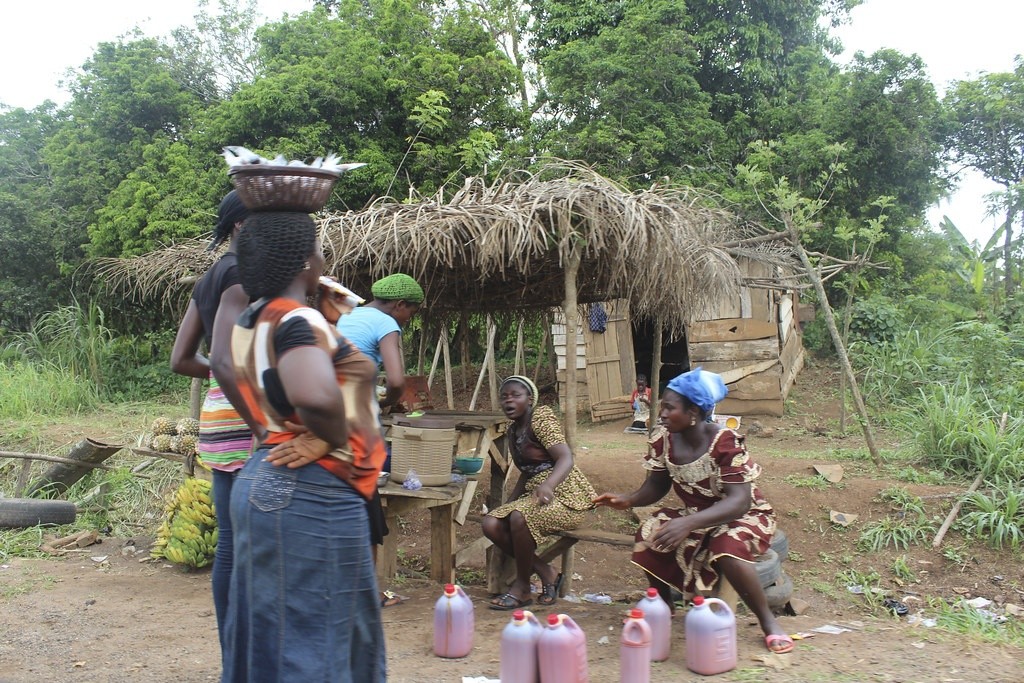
[454,457,483,475]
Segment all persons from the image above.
[630,374,651,429]
[482,375,599,610]
[170,188,387,683]
[336,274,424,608]
[592,367,795,654]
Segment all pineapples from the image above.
[144,416,200,455]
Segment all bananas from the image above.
[148,478,221,568]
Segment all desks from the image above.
[375,408,511,584]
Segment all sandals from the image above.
[378,596,403,608]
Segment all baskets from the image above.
[227,164,341,214]
[455,457,483,474]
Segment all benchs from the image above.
[486,527,739,621]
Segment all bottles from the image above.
[619,610,652,683]
[685,596,738,675]
[499,610,543,683]
[538,612,589,683]
[632,586,672,663]
[432,584,477,659]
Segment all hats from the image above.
[235,209,315,299]
[372,274,424,301]
[498,375,539,410]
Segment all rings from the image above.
[544,496,549,500]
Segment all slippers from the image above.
[536,573,565,605]
[763,635,795,654]
[622,612,676,625]
[489,593,533,610]
